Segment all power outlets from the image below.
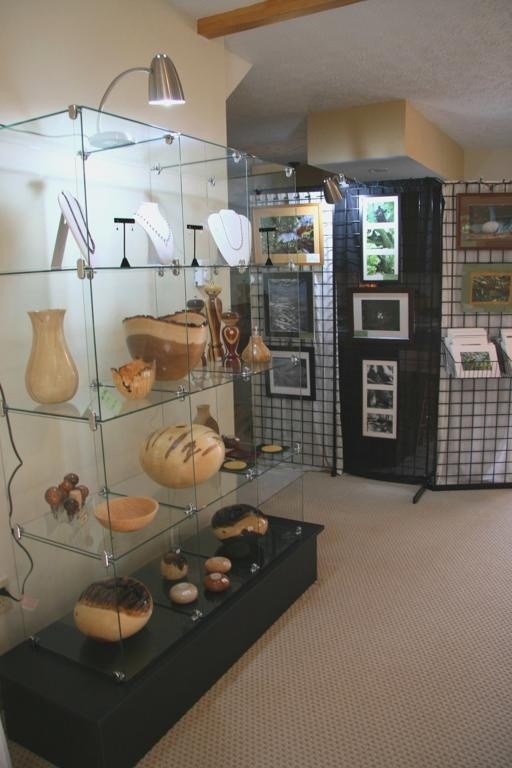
[0,578,16,614]
[195,259,211,287]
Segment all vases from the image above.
[192,405,219,435]
[25,308,78,404]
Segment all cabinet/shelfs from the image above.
[0,104,305,680]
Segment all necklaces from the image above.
[61,192,96,254]
[219,214,243,250]
[134,212,171,243]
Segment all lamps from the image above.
[321,175,350,204]
[89,51,186,150]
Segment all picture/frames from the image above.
[264,344,316,401]
[252,203,324,266]
[444,326,512,379]
[358,194,402,284]
[263,272,314,338]
[462,263,512,313]
[359,357,400,444]
[345,286,416,346]
[456,193,512,250]
